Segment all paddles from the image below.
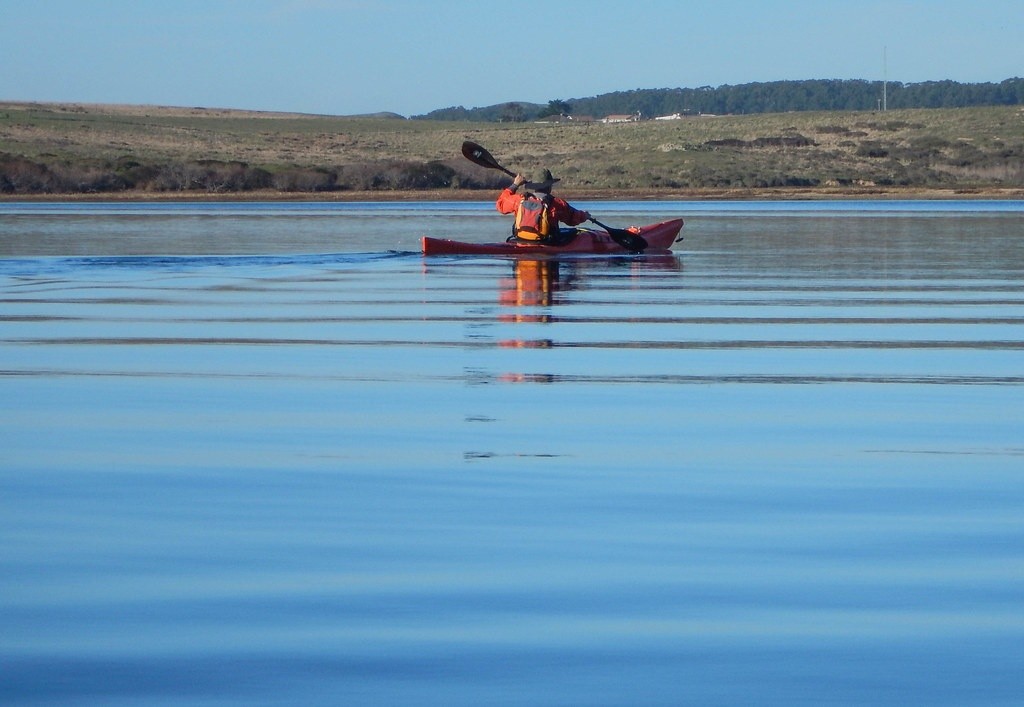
[458,137,651,253]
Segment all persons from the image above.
[496,168,590,246]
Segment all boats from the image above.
[423,218,685,255]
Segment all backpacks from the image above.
[514,198,552,242]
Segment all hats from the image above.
[526,167,561,189]
